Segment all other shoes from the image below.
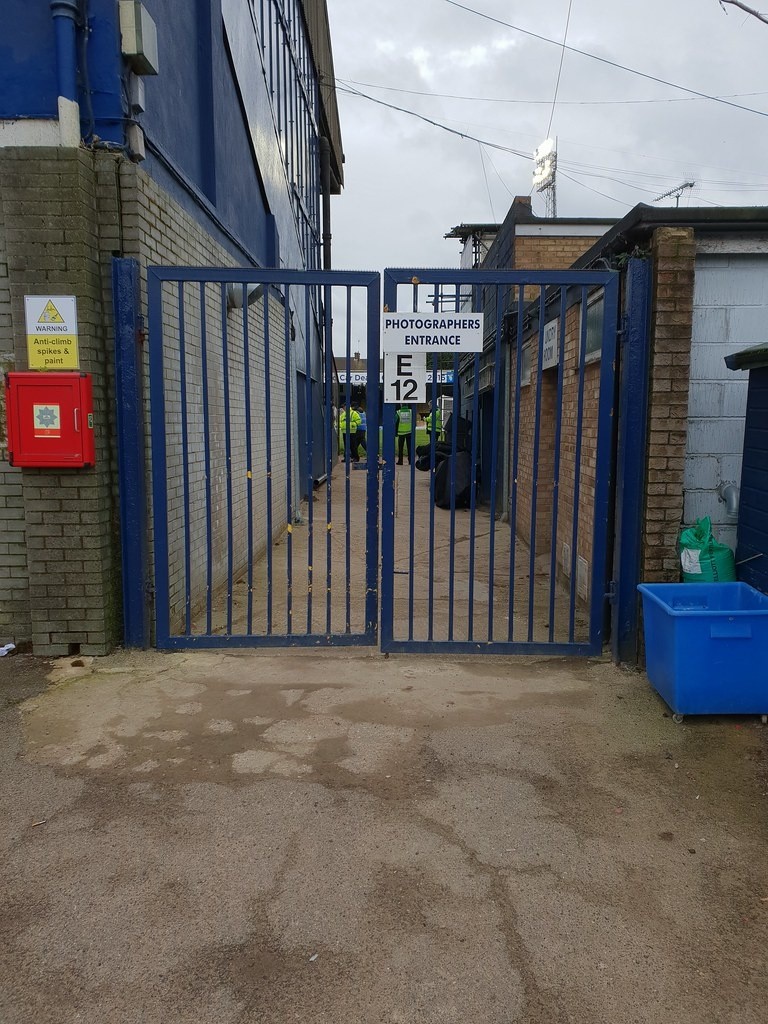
[341,458,344,463]
[395,462,402,466]
[353,457,360,462]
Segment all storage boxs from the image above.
[636,581,768,723]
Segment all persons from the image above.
[336,401,442,464]
